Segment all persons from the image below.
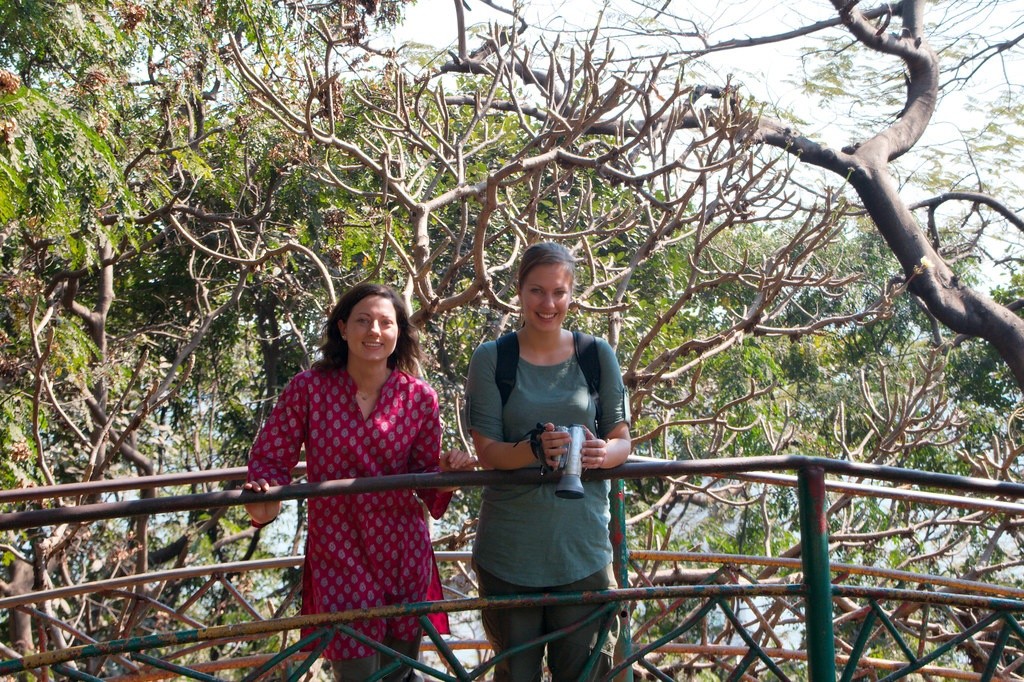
[464,243,631,682]
[243,283,477,682]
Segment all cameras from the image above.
[551,423,587,498]
